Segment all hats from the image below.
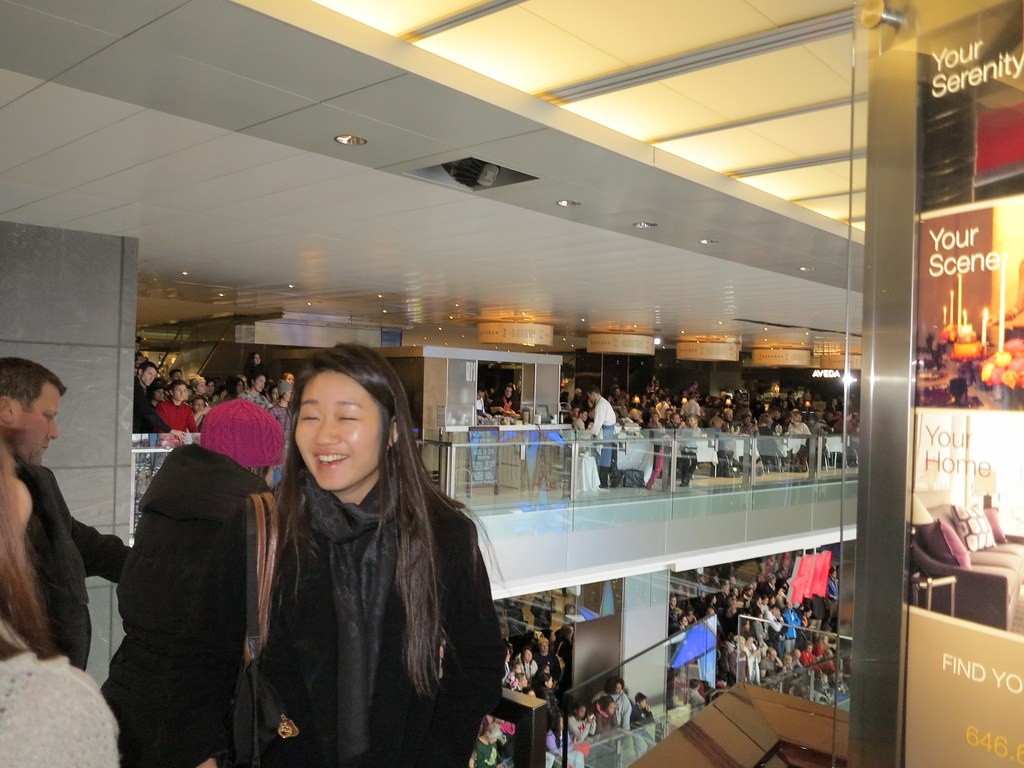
[278,380,293,395]
[188,373,206,388]
[201,398,286,469]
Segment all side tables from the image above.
[910,574,956,616]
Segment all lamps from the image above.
[675,336,740,362]
[752,344,811,367]
[820,352,862,370]
[586,327,656,356]
[477,316,554,347]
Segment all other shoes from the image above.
[608,484,621,488]
[598,483,608,489]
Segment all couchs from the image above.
[911,503,1024,633]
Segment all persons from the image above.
[0,353,134,768]
[181,341,507,768]
[476,374,862,490]
[468,551,847,768]
[135,335,295,489]
[99,398,286,768]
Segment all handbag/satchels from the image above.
[222,492,300,765]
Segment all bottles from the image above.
[736,426,740,433]
[731,426,734,434]
[775,426,782,435]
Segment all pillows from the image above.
[915,518,972,570]
[950,504,998,552]
[984,507,1008,543]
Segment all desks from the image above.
[584,433,850,488]
[431,423,572,498]
